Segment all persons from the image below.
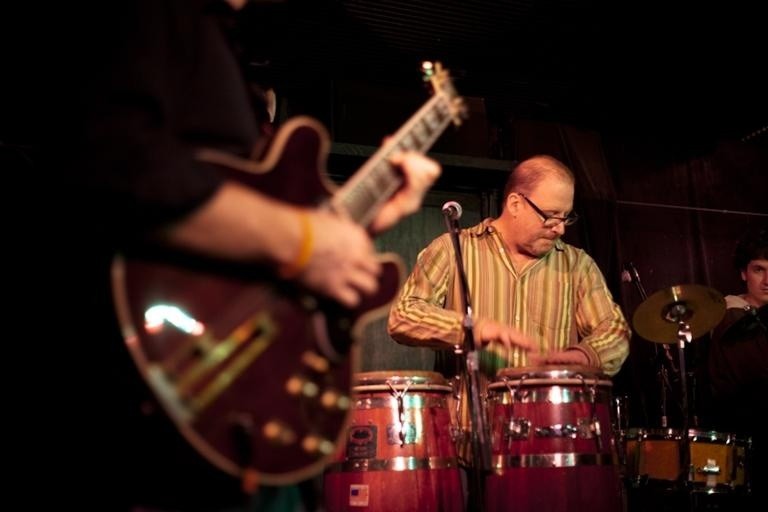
[1,0,441,510]
[386,156,633,374]
[706,241,767,349]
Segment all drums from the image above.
[486,369,621,511]
[628,430,732,496]
[325,371,463,512]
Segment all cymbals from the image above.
[634,283,726,344]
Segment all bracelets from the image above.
[282,209,315,278]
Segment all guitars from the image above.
[113,61,466,491]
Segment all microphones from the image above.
[442,201,464,221]
[621,261,640,283]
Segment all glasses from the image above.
[517,192,578,229]
